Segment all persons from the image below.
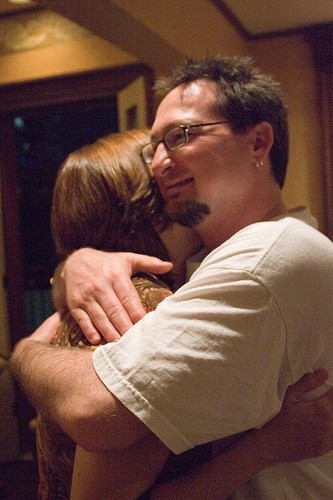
[35,129,333,500]
[10,50,333,500]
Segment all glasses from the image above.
[139,119,258,164]
